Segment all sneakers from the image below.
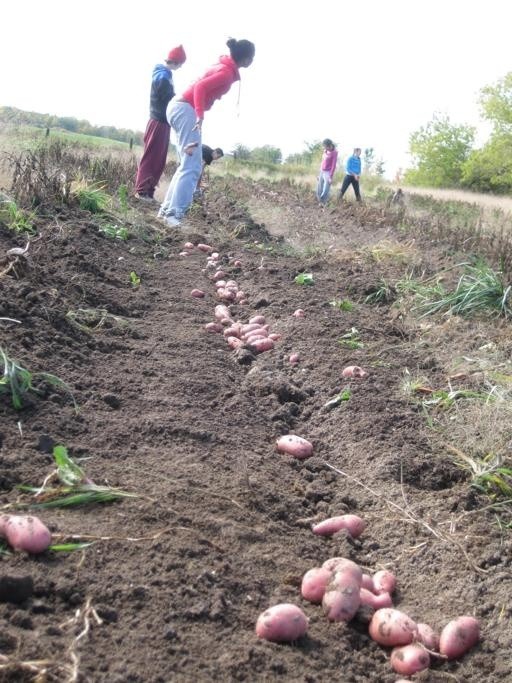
[134,193,153,203]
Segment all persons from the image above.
[337,147,362,202]
[315,138,338,203]
[135,45,187,205]
[201,143,224,176]
[157,38,256,227]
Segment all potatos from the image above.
[275,435,313,458]
[179,241,306,363]
[0,514,52,554]
[311,514,367,537]
[342,365,368,378]
[256,558,480,674]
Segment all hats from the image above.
[167,45,186,63]
